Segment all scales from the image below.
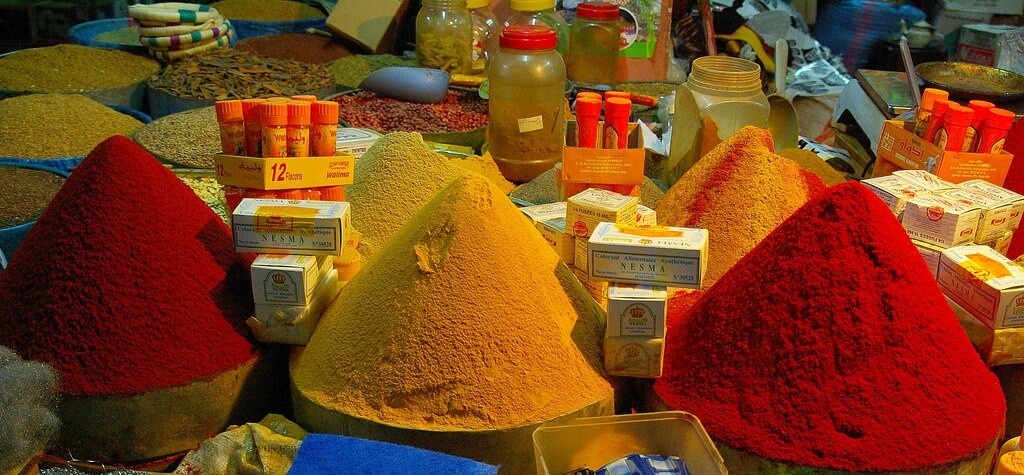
[832,61,1024,158]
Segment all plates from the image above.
[916,61,1022,108]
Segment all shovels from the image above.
[766,38,798,150]
[358,66,489,103]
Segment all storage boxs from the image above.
[932,0,1024,68]
[531,411,730,475]
[325,0,409,55]
[859,119,1024,366]
[215,127,381,345]
[509,118,712,377]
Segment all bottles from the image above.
[489,25,566,180]
[667,56,771,189]
[416,0,620,87]
